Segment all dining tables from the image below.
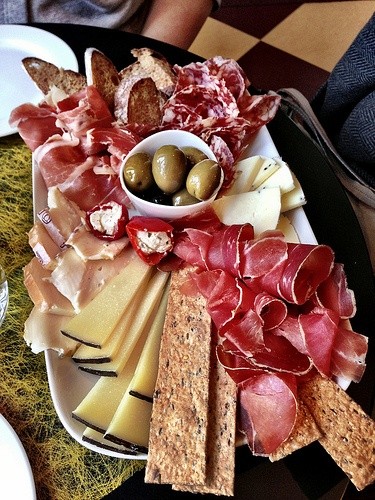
[1,19,375,500]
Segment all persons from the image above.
[1,0,223,51]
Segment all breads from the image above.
[21,47,176,132]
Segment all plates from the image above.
[0,24,79,138]
[32,123,353,459]
[1,414,36,500]
